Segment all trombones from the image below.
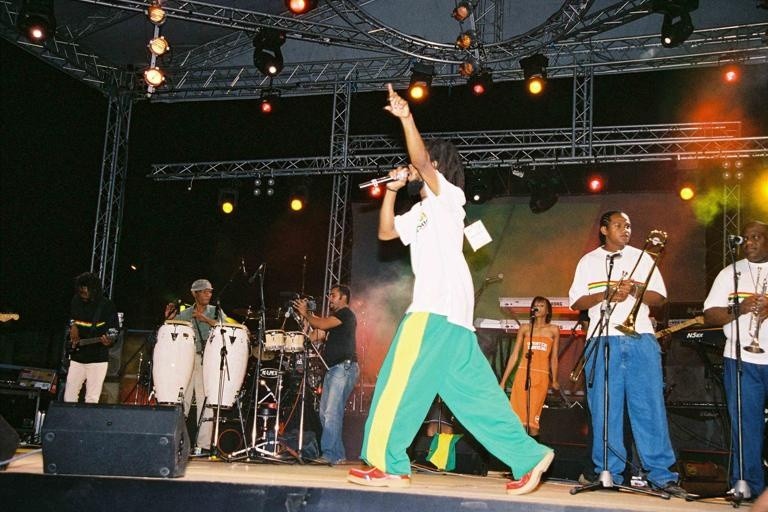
[568,230,667,383]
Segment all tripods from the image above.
[189,319,333,466]
[570,261,669,499]
[685,246,755,509]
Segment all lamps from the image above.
[17,0,60,45]
[286,0,319,15]
[651,1,700,48]
[451,0,478,79]
[519,54,549,98]
[530,189,558,213]
[256,86,282,116]
[142,0,169,97]
[252,27,286,77]
[469,67,493,95]
[407,63,435,104]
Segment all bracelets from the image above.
[633,287,638,298]
[386,187,398,192]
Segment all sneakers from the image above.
[348,467,411,488]
[653,481,687,497]
[314,458,345,465]
[506,451,554,495]
[578,472,597,484]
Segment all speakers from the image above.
[538,408,594,483]
[100,329,156,404]
[0,416,22,471]
[660,300,733,407]
[41,401,191,478]
[415,436,488,478]
[665,404,736,452]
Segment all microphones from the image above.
[729,234,744,245]
[359,172,409,190]
[248,264,263,284]
[169,299,182,315]
[532,307,540,314]
[241,256,247,277]
[606,252,623,260]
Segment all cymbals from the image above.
[235,306,264,319]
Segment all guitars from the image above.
[651,316,705,343]
[46,332,117,366]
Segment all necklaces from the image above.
[748,260,767,294]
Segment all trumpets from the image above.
[742,266,768,354]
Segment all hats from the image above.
[191,279,213,291]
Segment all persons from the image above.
[703,221,768,501]
[164,279,228,459]
[292,285,361,465]
[64,273,119,403]
[499,295,560,442]
[568,211,687,498]
[348,83,554,494]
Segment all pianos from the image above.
[498,296,585,337]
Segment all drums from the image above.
[152,320,196,406]
[283,331,305,353]
[203,322,250,409]
[262,329,285,351]
